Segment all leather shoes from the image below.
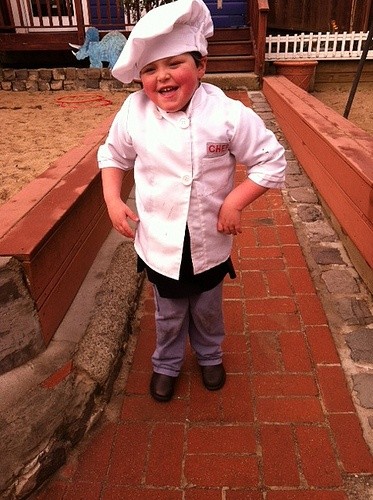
[150,372,175,402]
[202,364,226,391]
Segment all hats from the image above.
[111,1,213,84]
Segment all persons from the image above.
[97,0,286,402]
